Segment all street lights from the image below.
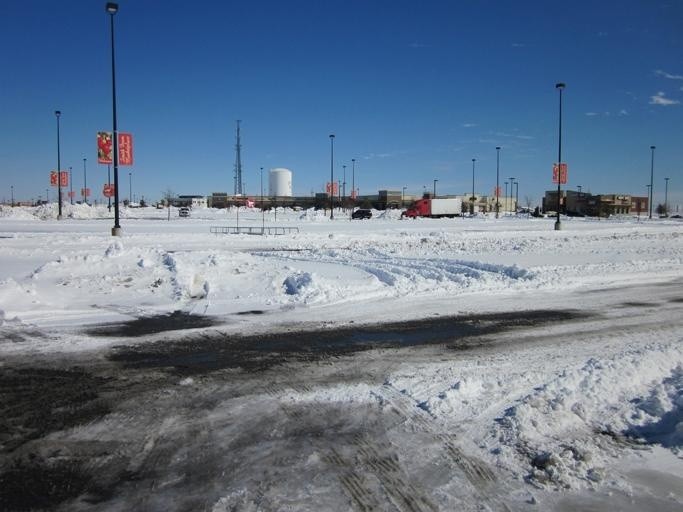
[326,134,355,221]
[432,179,437,198]
[471,158,476,203]
[104,1,123,238]
[494,147,501,219]
[552,82,567,229]
[644,144,670,219]
[69,156,133,205]
[53,108,63,223]
[504,176,519,217]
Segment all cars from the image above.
[352,210,372,221]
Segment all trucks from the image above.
[400,198,463,219]
